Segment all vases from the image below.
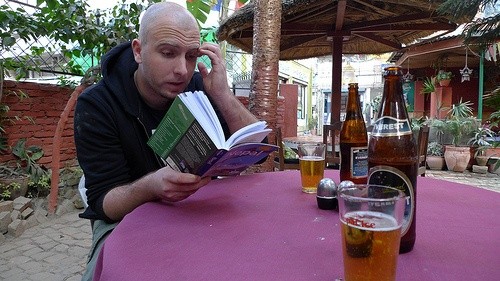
[488,164,498,173]
[0,175,29,200]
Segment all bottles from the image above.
[339,83,368,185]
[368,66,417,252]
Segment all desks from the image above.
[284,135,340,157]
[94,171,500,281]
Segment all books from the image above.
[147,90,280,178]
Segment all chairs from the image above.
[273,127,300,171]
[418,127,430,177]
[323,125,340,167]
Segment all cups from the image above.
[337,185,405,281]
[297,143,326,194]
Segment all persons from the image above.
[73,1,269,281]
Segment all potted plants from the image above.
[444,97,480,173]
[436,70,452,86]
[467,124,497,166]
[418,76,447,170]
